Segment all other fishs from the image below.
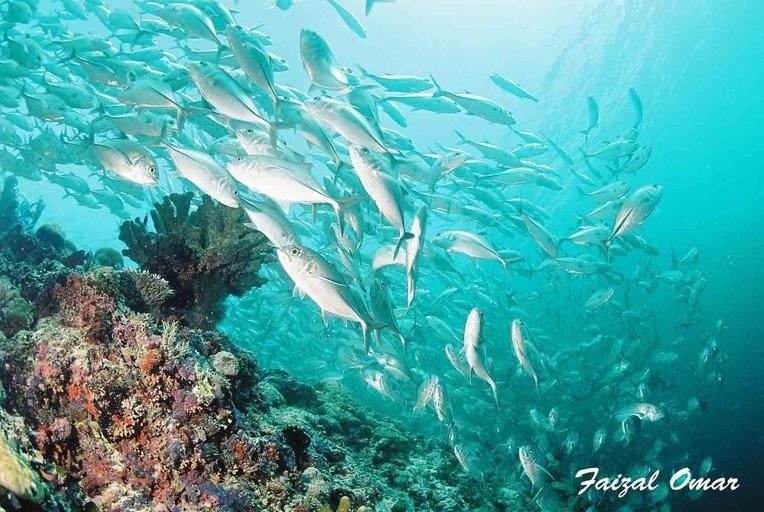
[0,1,728,512]
